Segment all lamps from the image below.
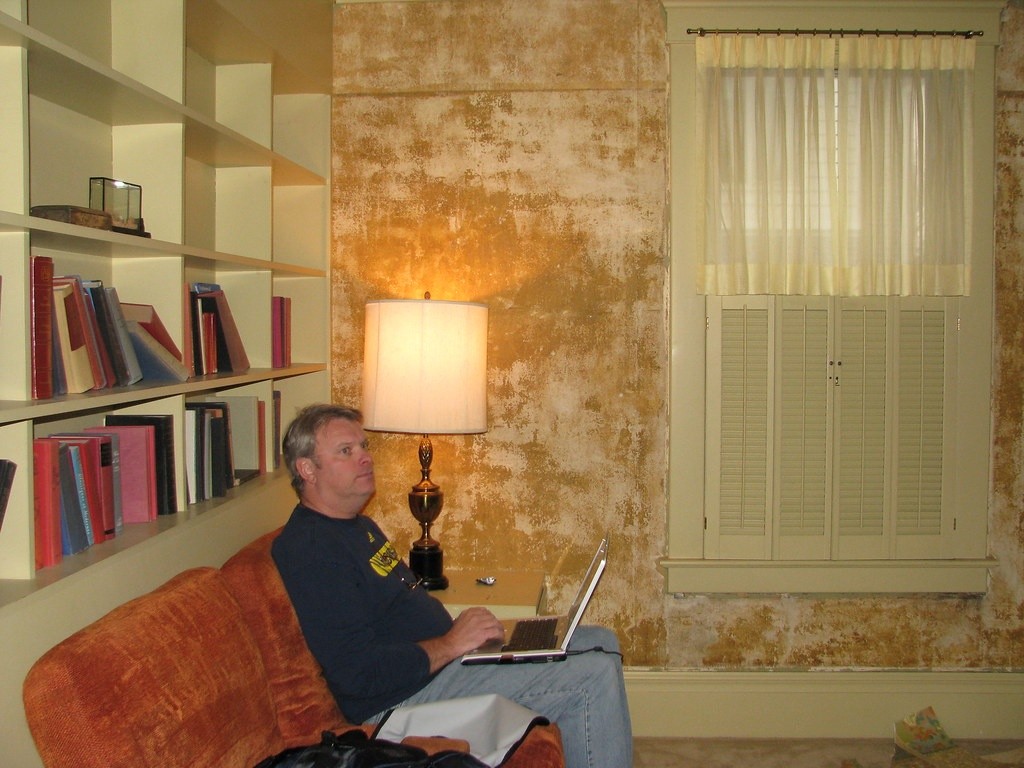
[357,292,489,591]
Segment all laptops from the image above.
[459,532,610,665]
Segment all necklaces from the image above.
[367,537,418,591]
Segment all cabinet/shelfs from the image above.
[0,0,334,768]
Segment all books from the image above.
[34,415,177,570]
[29,256,191,398]
[272,297,292,368]
[185,396,267,504]
[184,283,250,376]
[0,459,16,529]
[272,390,280,469]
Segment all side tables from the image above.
[426,570,549,621]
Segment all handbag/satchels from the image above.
[252,694,550,768]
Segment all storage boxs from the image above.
[88,176,142,235]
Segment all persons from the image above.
[270,403,632,768]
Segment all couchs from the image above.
[21,523,565,768]
